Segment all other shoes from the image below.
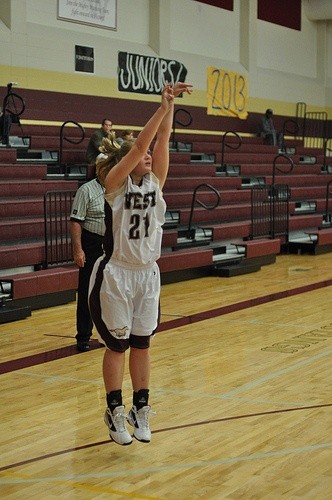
[76,343,89,352]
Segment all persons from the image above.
[69,158,106,352]
[116,129,133,146]
[255,109,283,146]
[87,82,193,445]
[86,118,113,164]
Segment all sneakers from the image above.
[104,405,133,446]
[126,404,157,443]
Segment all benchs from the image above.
[0,121,332,311]
